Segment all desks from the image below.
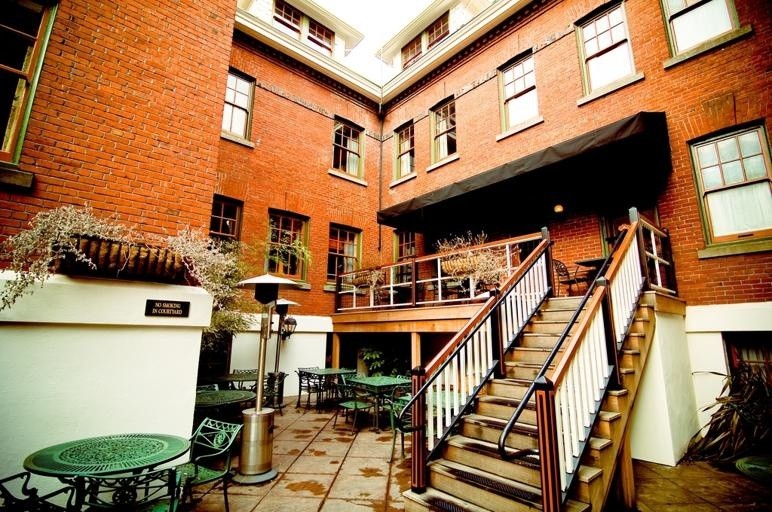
[575,257,614,278]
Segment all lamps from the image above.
[281,316,298,340]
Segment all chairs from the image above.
[194,368,289,464]
[552,258,595,296]
[430,274,456,306]
[1,418,246,512]
[295,366,480,463]
